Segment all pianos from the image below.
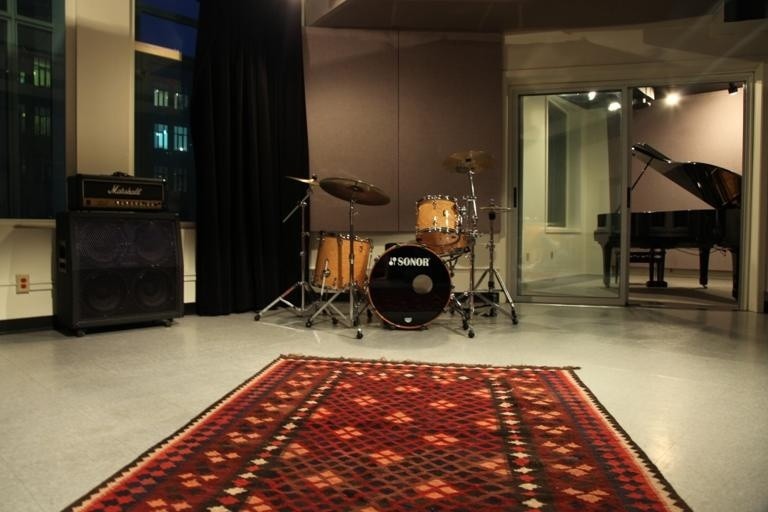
[594,143,741,297]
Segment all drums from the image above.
[416,196,461,246]
[311,234,371,293]
[369,246,451,328]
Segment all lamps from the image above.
[606,95,621,112]
[728,82,738,94]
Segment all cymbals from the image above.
[481,205,511,213]
[443,150,494,175]
[318,177,389,206]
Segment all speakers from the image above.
[724,1,768,22]
[55,210,184,337]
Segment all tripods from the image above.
[306,206,372,339]
[459,219,518,324]
[254,202,338,326]
[449,168,518,338]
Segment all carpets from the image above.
[60,354,696,511]
